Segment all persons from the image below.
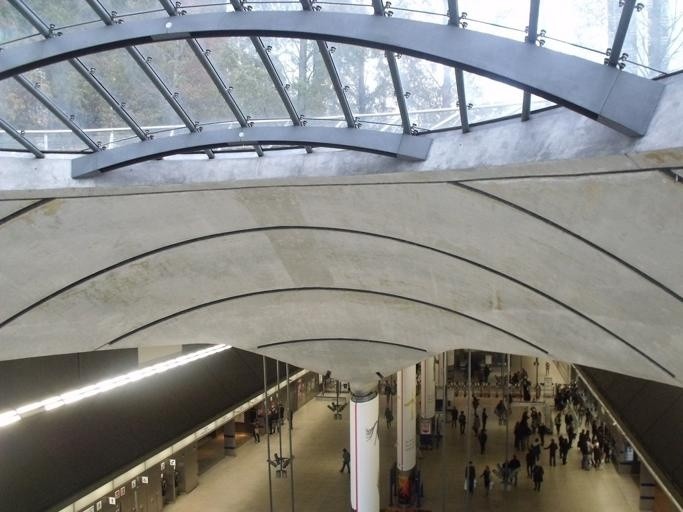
[384,406,394,429]
[382,374,421,401]
[443,360,613,495]
[339,446,350,475]
[243,396,287,444]
